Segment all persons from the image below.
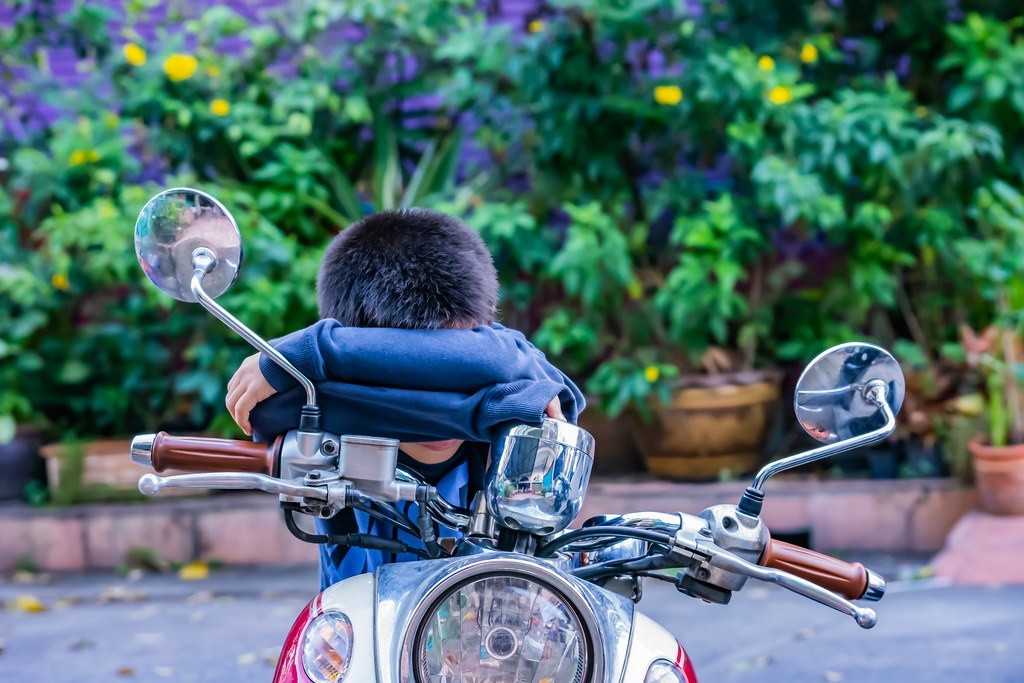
[225,208,587,593]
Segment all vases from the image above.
[968,434,1024,515]
[623,383,779,480]
[39,439,209,496]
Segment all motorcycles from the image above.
[130,186,905,682]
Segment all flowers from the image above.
[0,0,1024,445]
